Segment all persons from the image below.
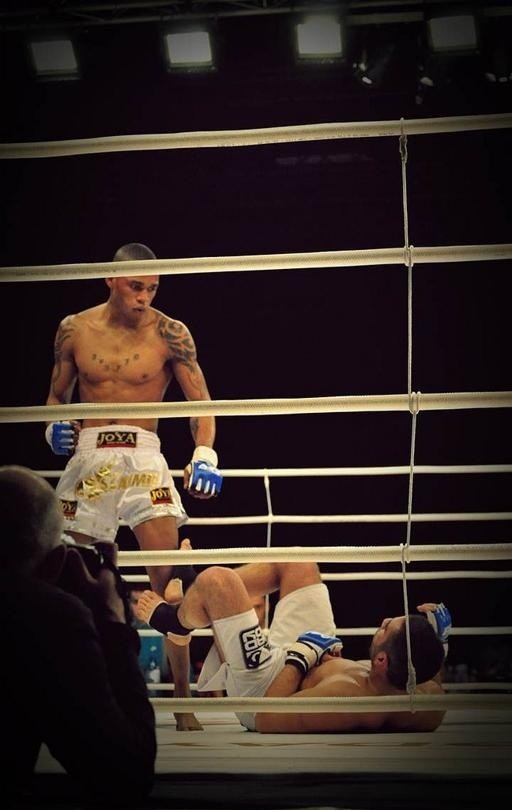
[140,535,455,735]
[41,243,224,731]
[1,466,161,810]
[210,595,267,697]
[123,588,197,698]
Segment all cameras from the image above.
[60,542,102,596]
